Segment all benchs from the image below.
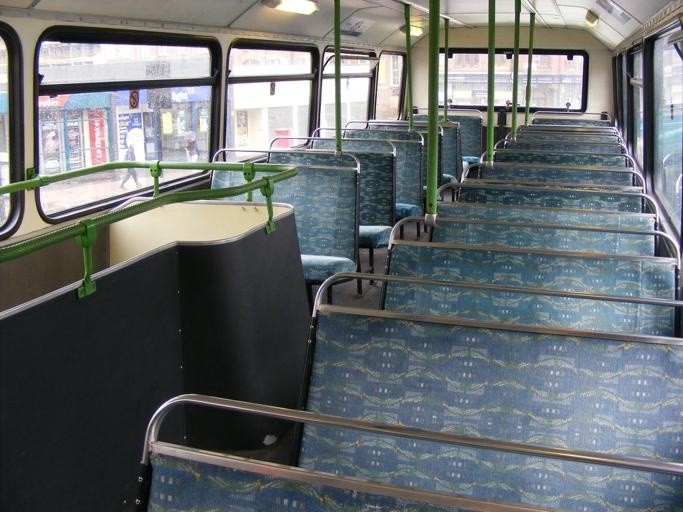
[120,110,683,512]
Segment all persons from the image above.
[185,129,200,174]
[116,141,141,190]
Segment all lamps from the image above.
[585,11,599,28]
[398,23,424,38]
[261,0,322,17]
